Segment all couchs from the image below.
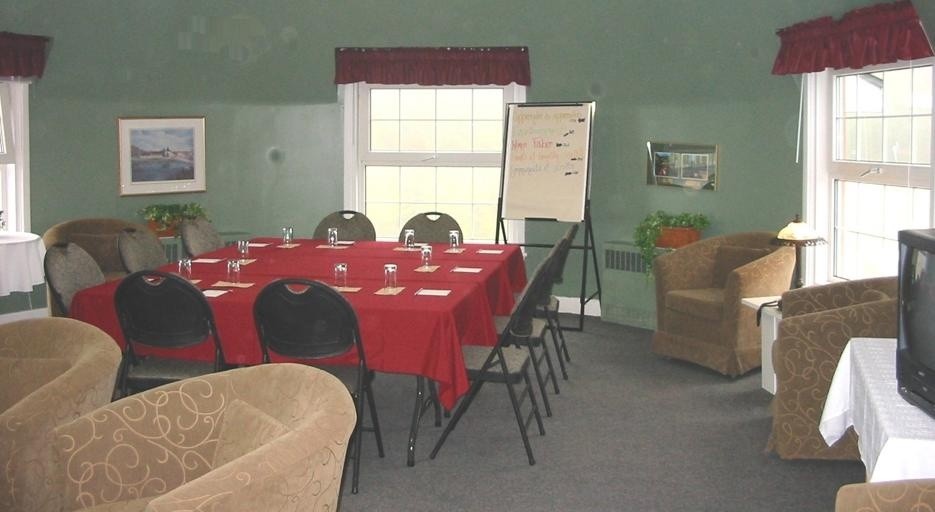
[41,218,131,319]
[831,480,935,512]
[52,361,356,512]
[771,278,900,461]
[654,230,797,379]
[1,319,121,511]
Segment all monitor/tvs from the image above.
[894,228,935,418]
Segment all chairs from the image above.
[111,269,229,400]
[184,220,224,257]
[45,238,106,313]
[493,237,570,417]
[513,222,581,380]
[399,212,464,244]
[252,279,385,494]
[313,212,376,241]
[430,261,545,465]
[118,223,170,272]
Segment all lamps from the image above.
[771,215,826,307]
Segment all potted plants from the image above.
[635,210,709,285]
[142,204,207,237]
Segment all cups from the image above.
[449,230,459,252]
[328,228,337,247]
[227,259,240,285]
[178,258,192,281]
[238,241,249,259]
[406,229,414,248]
[334,262,347,289]
[421,246,432,271]
[384,264,397,293]
[281,227,294,247]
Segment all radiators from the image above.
[160,231,250,264]
[599,240,677,332]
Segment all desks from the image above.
[69,236,530,466]
[742,296,783,394]
[821,337,934,482]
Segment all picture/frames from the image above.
[644,142,719,194]
[118,115,208,195]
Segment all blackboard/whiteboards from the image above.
[500,103,591,223]
